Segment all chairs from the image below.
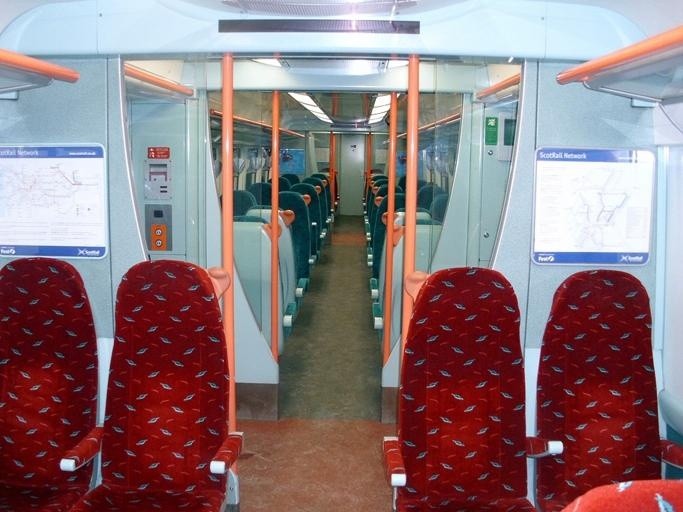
[362,169,451,351]
[530,267,683,512]
[0,256,103,510]
[382,264,539,512]
[222,166,340,356]
[562,478,683,512]
[70,257,242,511]
